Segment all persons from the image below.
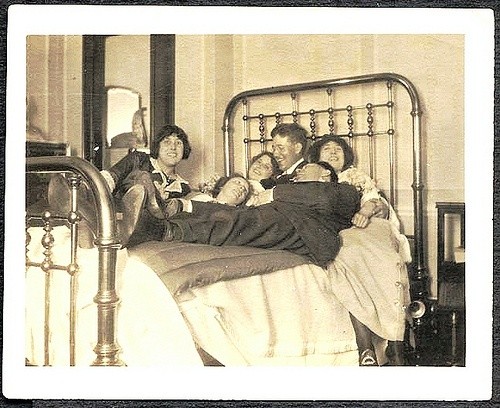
[135,123,310,219]
[45,160,362,268]
[308,132,413,367]
[26,125,192,229]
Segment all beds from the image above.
[26,73,431,367]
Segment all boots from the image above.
[48,175,98,249]
[116,184,167,249]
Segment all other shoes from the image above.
[358,347,378,366]
[137,172,181,219]
[384,341,408,366]
